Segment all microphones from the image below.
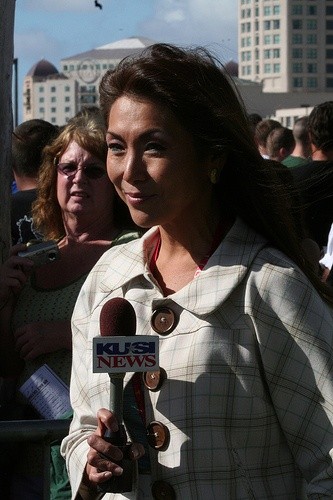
[93,297,160,494]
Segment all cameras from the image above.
[18,240,60,272]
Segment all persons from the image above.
[0,106,149,500]
[60,43,333,500]
[247,100,333,286]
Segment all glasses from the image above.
[56,162,105,180]
